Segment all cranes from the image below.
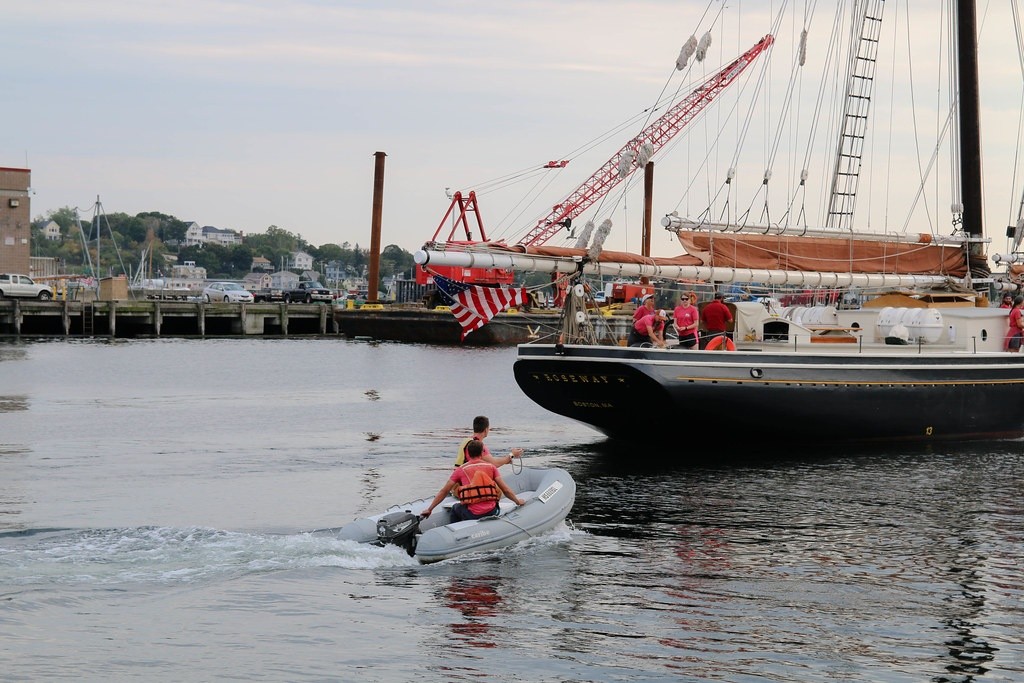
[417,34,774,285]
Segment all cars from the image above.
[332,288,388,299]
[202,282,254,303]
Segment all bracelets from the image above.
[685,326,687,330]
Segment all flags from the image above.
[433,274,528,341]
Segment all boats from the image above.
[334,151,671,345]
[338,467,576,566]
[127,240,190,296]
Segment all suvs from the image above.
[0,273,53,302]
[283,281,333,304]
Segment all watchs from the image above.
[509,452,515,458]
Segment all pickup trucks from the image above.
[245,287,282,303]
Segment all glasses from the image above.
[681,298,689,301]
[648,297,653,299]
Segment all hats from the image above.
[642,294,654,304]
[656,309,666,320]
[715,292,726,299]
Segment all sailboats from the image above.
[416,1,1024,440]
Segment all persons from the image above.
[1005,295,1024,352]
[701,292,733,340]
[627,294,668,346]
[674,292,699,350]
[452,416,524,497]
[420,442,525,523]
[1000,293,1013,308]
